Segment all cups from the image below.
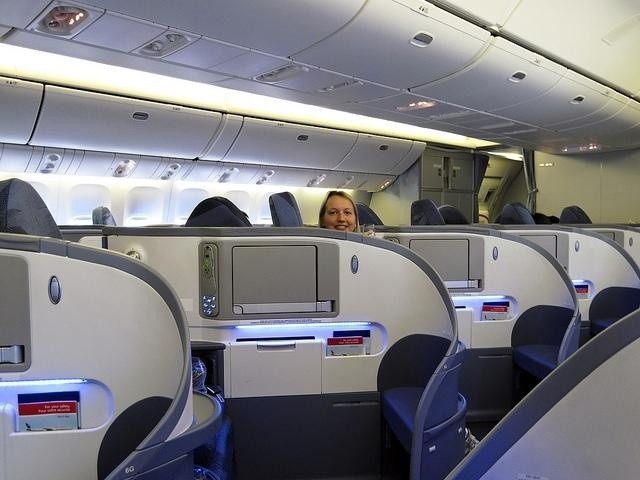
[364,223,377,238]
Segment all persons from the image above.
[319,190,376,239]
